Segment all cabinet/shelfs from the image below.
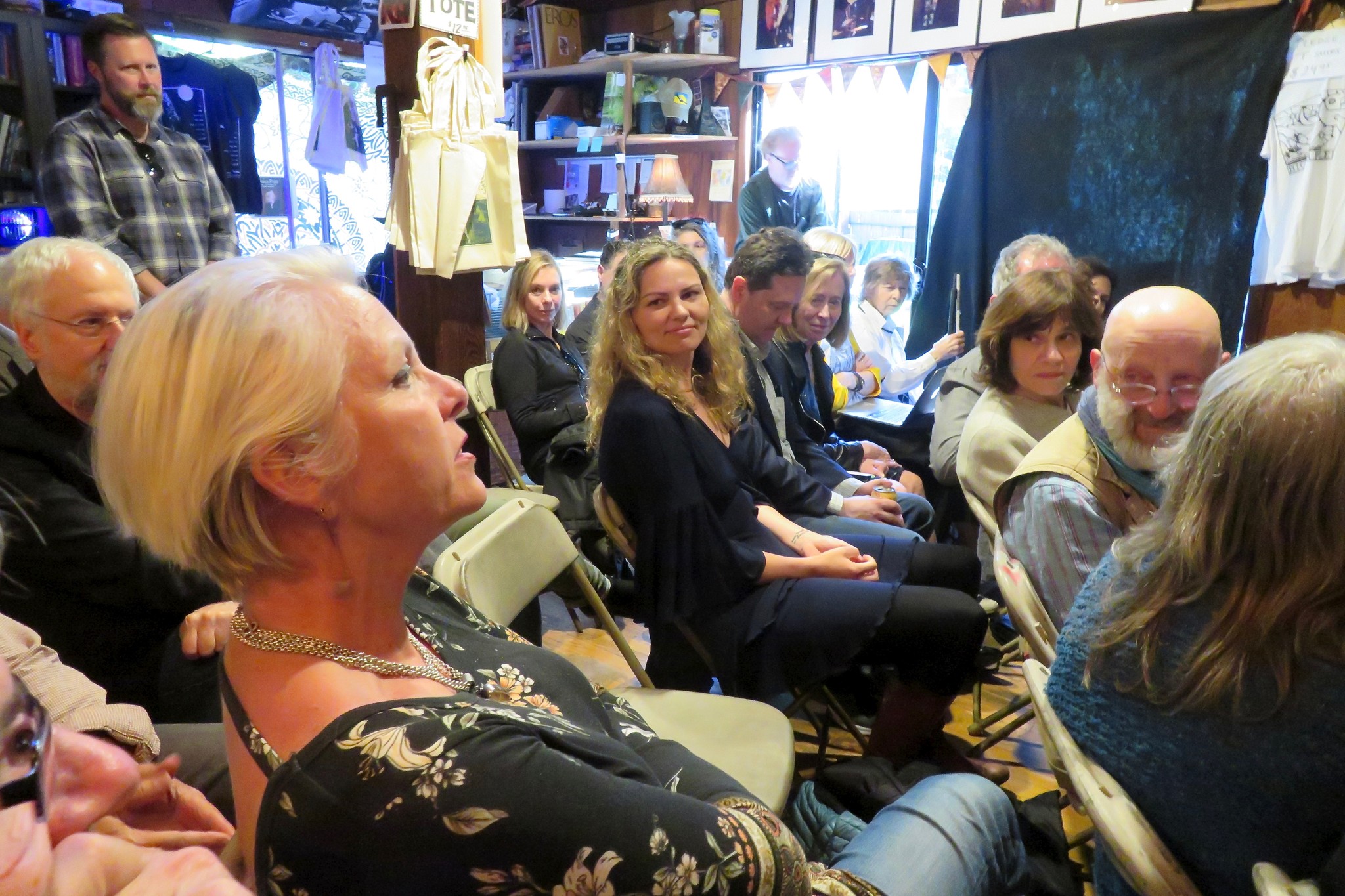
[0,8,100,238]
[504,52,736,222]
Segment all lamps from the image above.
[638,153,694,240]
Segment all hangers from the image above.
[194,40,231,64]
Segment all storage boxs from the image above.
[604,32,659,55]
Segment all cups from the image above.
[544,189,566,215]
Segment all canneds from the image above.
[871,485,897,503]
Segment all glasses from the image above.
[771,153,799,169]
[560,347,585,376]
[1100,350,1201,410]
[0,675,54,821]
[671,217,709,230]
[136,141,165,182]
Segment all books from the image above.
[0,24,25,86]
[527,4,580,72]
[44,30,89,88]
[0,111,55,249]
[503,79,534,141]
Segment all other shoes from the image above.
[977,595,999,615]
[977,645,1003,664]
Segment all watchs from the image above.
[849,371,865,392]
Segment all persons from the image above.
[36,14,242,307]
[0,234,242,721]
[0,611,258,896]
[490,217,1345,896]
[734,127,836,263]
[757,0,793,49]
[88,247,1086,896]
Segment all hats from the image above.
[657,78,693,123]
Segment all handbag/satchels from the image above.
[385,36,533,281]
[305,42,368,175]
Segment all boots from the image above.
[862,679,1010,786]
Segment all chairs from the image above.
[432,362,1320,896]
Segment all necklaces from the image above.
[230,603,475,692]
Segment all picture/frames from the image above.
[893,0,980,57]
[737,0,812,69]
[813,0,894,62]
[1080,0,1193,29]
[978,0,1079,44]
